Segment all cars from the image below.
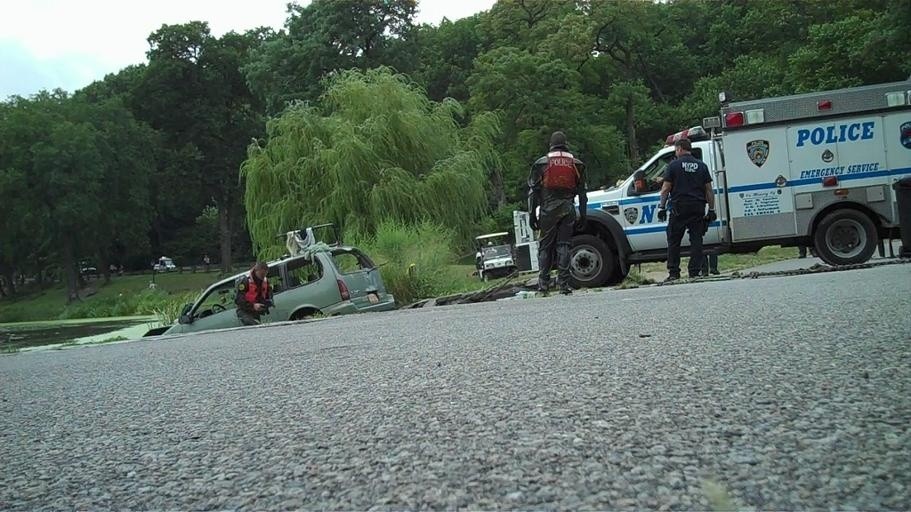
[152,257,177,272]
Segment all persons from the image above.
[798,245,809,259]
[233,261,276,326]
[702,254,721,276]
[527,129,591,294]
[658,138,718,283]
[634,170,664,190]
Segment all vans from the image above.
[140,246,396,338]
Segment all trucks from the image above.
[538,80,911,289]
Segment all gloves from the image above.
[656,204,667,222]
[529,212,542,231]
[703,209,718,223]
[574,215,589,232]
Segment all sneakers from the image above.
[687,272,703,282]
[536,287,552,299]
[559,286,575,296]
[662,272,682,285]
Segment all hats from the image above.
[548,131,569,150]
